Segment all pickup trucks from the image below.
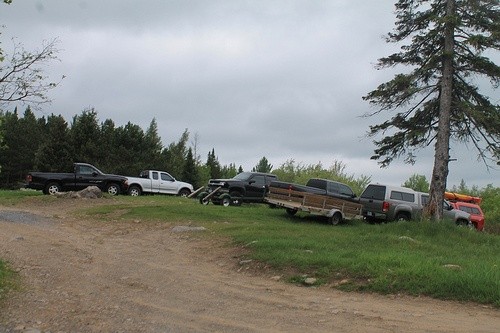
[121,170,195,197]
[266,178,363,224]
[358,182,471,227]
[24,163,127,197]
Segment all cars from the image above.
[200,170,280,206]
